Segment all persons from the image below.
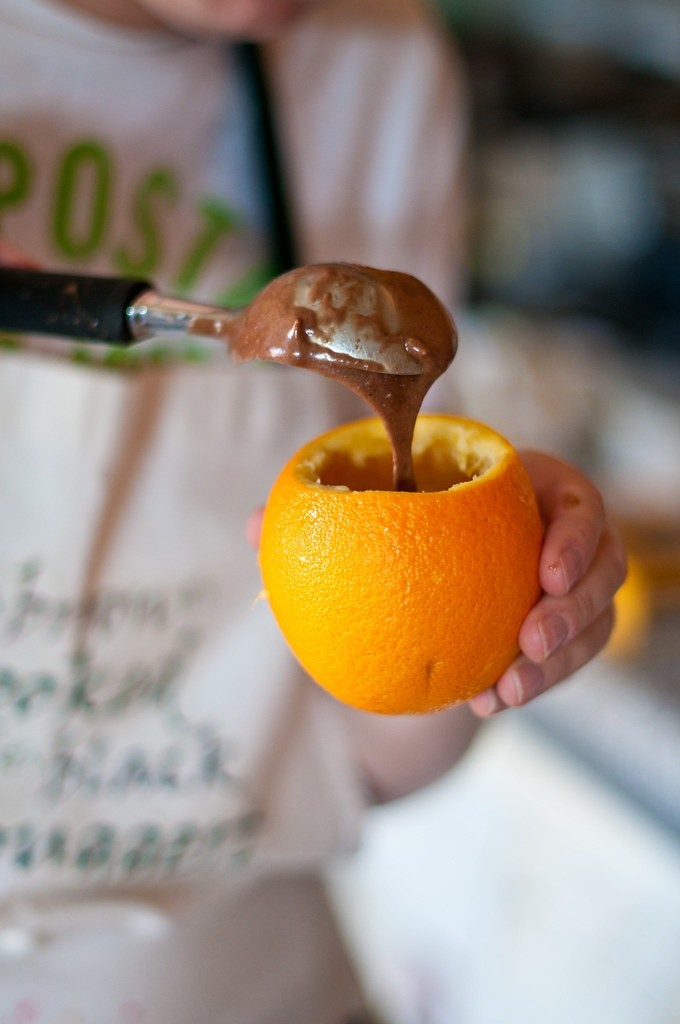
[1,2,630,1024]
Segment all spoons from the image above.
[0,262,458,376]
